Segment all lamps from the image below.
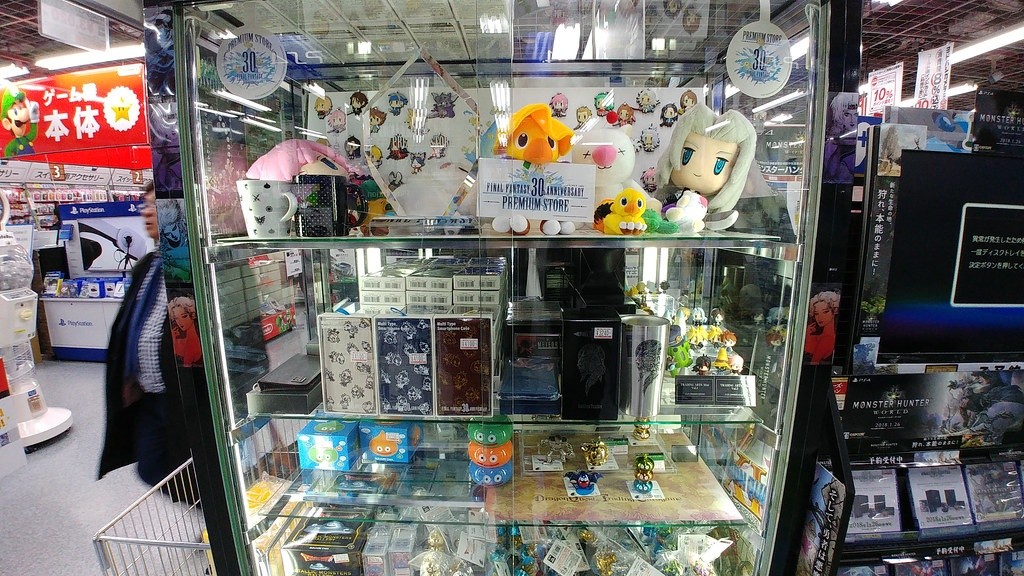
[0,39,147,75]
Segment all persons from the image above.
[96,180,203,509]
[804,290,841,366]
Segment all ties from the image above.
[124,260,162,378]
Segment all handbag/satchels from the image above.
[257,352,321,391]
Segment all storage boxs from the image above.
[245,248,626,576]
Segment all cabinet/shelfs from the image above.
[169,0,832,576]
[795,348,1024,576]
[39,298,125,363]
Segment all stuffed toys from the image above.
[245,102,757,236]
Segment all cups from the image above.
[618,315,670,418]
[291,174,368,237]
[235,179,298,239]
